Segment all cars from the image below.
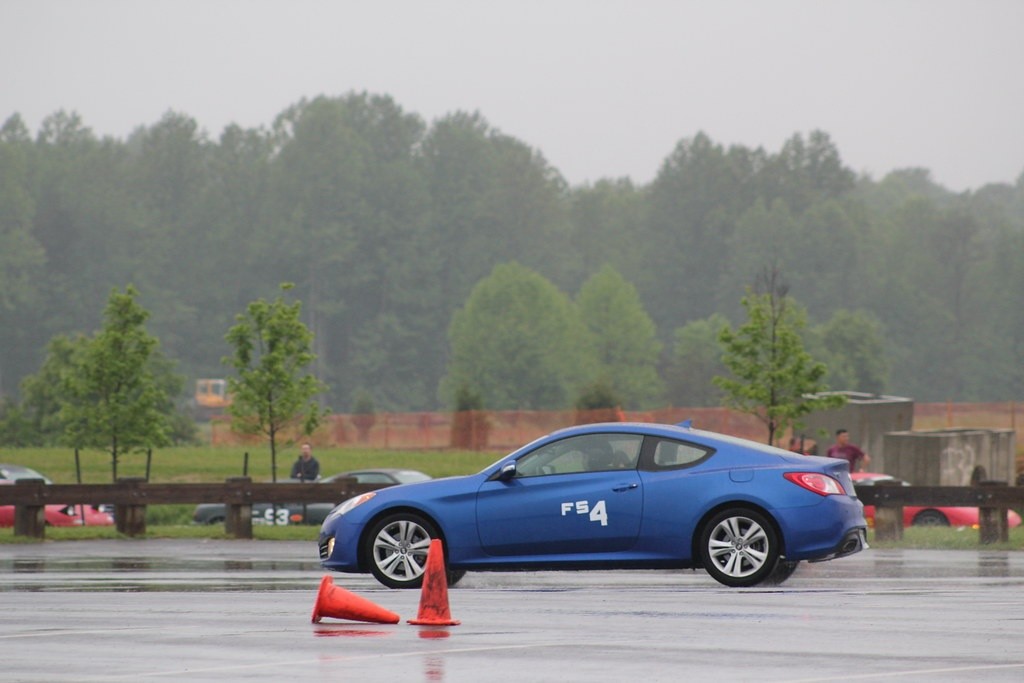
[0,464,115,530]
[846,472,1022,529]
[191,467,435,526]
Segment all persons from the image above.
[291,442,319,480]
[826,429,871,473]
[576,440,613,472]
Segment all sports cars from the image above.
[318,420,872,591]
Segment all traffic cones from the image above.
[312,574,401,626]
[406,539,462,626]
